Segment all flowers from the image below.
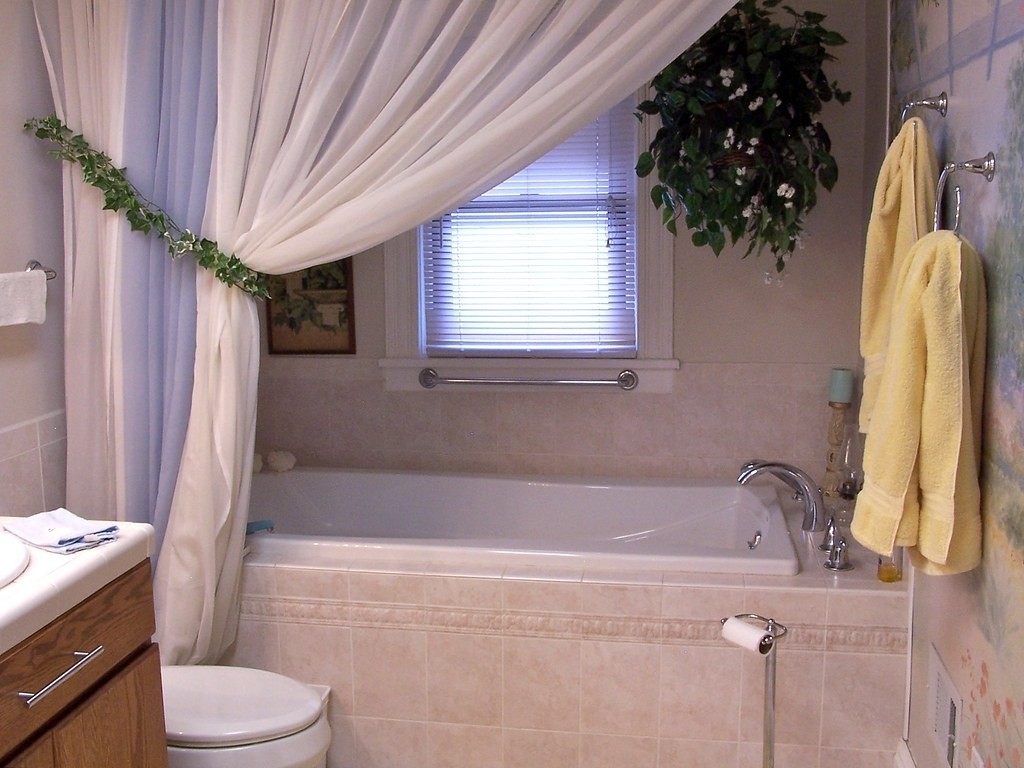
[633,2,852,274]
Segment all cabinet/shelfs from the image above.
[0,558,170,767]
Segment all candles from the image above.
[829,366,853,402]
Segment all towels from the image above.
[849,229,984,578]
[857,116,944,440]
[2,507,128,557]
[0,268,47,328]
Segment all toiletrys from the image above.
[872,538,904,583]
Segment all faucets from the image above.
[737,459,831,534]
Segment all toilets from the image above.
[160,662,332,768]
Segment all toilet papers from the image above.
[717,616,777,659]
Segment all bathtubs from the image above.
[171,451,923,768]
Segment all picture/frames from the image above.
[265,254,356,357]
